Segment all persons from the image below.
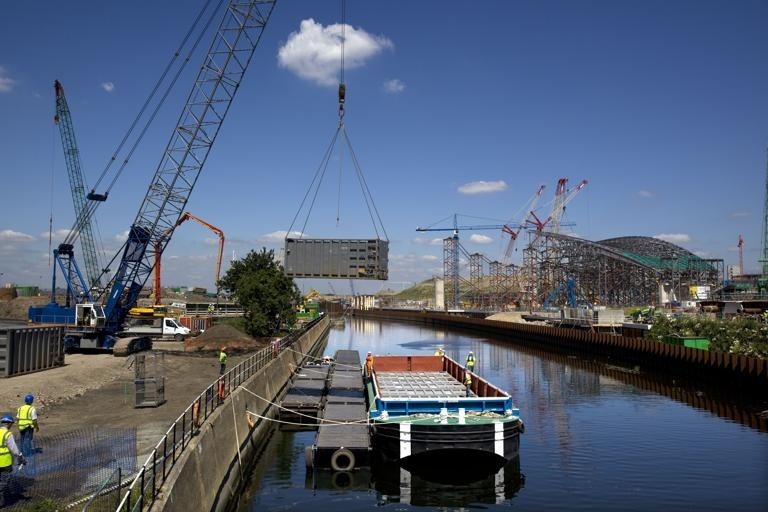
[464,368,472,397]
[14,395,39,460]
[465,351,476,371]
[218,346,227,379]
[366,351,373,378]
[207,303,215,315]
[0,414,28,509]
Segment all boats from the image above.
[366,354,524,447]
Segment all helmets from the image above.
[0,415,14,424]
[25,395,33,402]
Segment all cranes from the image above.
[415,177,588,260]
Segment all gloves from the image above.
[19,455,27,465]
[33,420,39,433]
